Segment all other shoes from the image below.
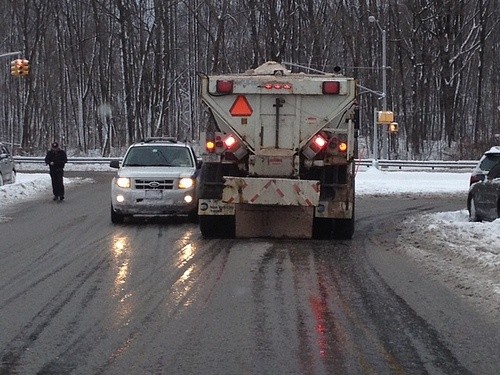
[60,196,64,200]
[53,196,58,200]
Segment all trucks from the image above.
[198,60,362,242]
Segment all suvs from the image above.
[0,140,17,191]
[109,137,202,225]
[466,146,500,223]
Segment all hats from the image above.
[52,143,58,148]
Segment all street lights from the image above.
[366,16,389,165]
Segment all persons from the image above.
[44,142,68,201]
